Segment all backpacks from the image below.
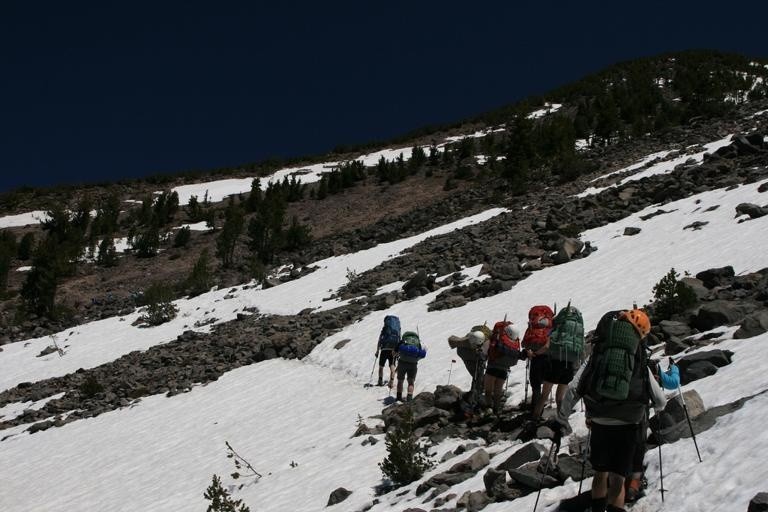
[489,319,522,364]
[383,315,401,344]
[402,331,420,357]
[578,308,652,423]
[554,308,584,353]
[523,306,554,353]
[457,323,491,361]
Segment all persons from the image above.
[449,324,493,414]
[557,309,666,512]
[484,320,520,418]
[375,316,400,388]
[391,332,426,402]
[522,306,555,418]
[529,305,583,422]
[605,336,680,500]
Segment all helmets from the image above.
[469,331,485,344]
[505,324,520,340]
[617,310,651,339]
